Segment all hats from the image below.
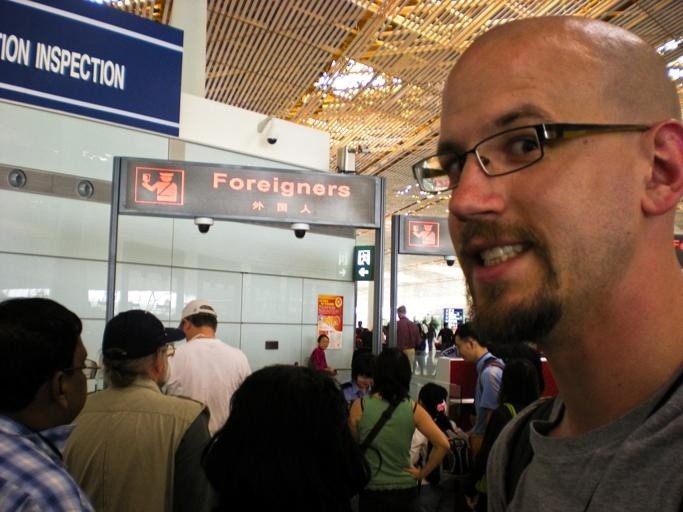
[178,299,217,331]
[103,310,185,358]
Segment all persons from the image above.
[307,305,542,512]
[158,298,252,437]
[0,298,98,512]
[61,309,215,512]
[200,364,374,512]
[410,16,683,512]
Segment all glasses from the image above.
[63,361,99,379]
[412,110,652,193]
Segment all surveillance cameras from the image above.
[193,217,213,235]
[267,127,278,144]
[290,224,310,238]
[445,256,455,267]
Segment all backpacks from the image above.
[479,358,544,413]
